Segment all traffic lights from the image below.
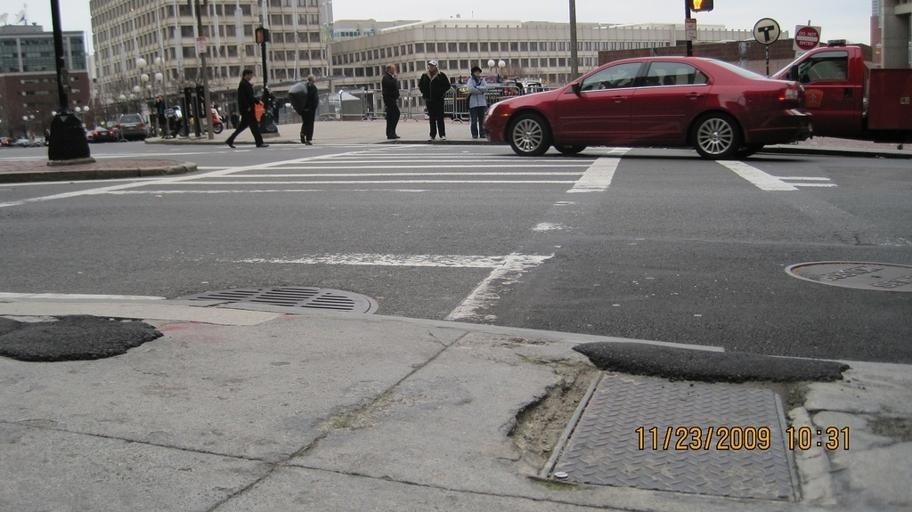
[688,0,712,11]
[255,27,265,46]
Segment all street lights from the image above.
[20,57,163,121]
[486,57,505,82]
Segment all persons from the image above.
[156,94,168,139]
[301,74,319,145]
[226,70,269,148]
[418,60,450,140]
[467,66,489,138]
[382,64,401,139]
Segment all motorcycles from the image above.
[165,84,293,138]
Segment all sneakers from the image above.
[224,141,236,149]
[300,132,312,146]
[386,135,401,139]
[255,144,270,148]
[428,136,446,141]
[471,133,487,139]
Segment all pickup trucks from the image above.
[766,40,910,149]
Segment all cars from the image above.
[346,81,545,117]
[482,56,805,164]
[1,114,151,146]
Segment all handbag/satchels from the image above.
[465,93,472,113]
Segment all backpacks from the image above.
[287,81,309,114]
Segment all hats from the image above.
[427,60,439,69]
